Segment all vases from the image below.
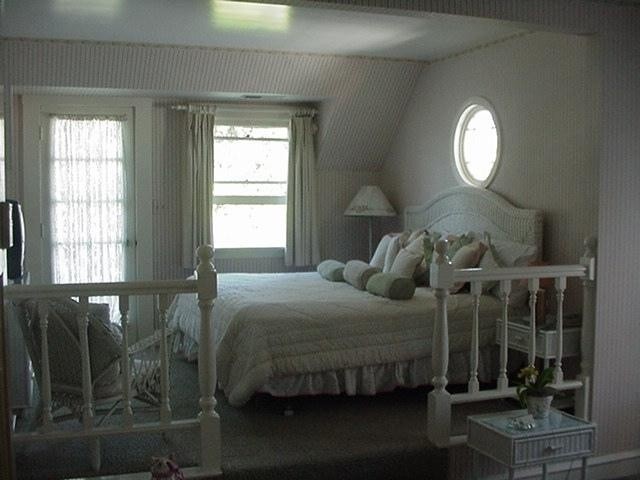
[523,394,550,416]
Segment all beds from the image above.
[161,181,542,417]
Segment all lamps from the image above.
[344,185,396,263]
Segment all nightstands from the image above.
[492,311,582,396]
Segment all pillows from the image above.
[315,229,535,304]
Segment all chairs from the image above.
[2,287,175,444]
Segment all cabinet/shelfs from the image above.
[467,410,596,480]
